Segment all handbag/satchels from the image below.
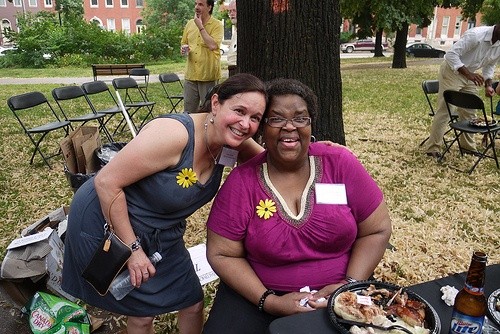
[80,190,132,296]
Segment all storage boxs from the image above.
[1,204,92,334]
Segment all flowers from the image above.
[177,167,197,188]
[255,199,278,219]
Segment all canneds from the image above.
[183,45,190,56]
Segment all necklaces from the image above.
[204,115,223,164]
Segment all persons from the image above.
[425,21,500,157]
[227,0,236,76]
[61,73,265,334]
[180,0,224,113]
[201,77,393,334]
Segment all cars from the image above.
[340,37,388,53]
[405,42,446,58]
[220,43,228,54]
[0,44,52,60]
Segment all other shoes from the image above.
[426,151,446,161]
[463,150,487,159]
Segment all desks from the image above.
[268,264,500,334]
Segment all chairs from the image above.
[419,81,500,174]
[7,68,156,170]
[159,73,185,113]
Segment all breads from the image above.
[334,292,378,322]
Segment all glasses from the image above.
[264,117,311,127]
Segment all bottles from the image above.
[107,252,163,300]
[449,251,490,334]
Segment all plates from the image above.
[327,279,441,334]
[469,117,498,128]
[487,287,500,328]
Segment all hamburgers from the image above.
[478,114,492,125]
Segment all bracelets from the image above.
[200,28,204,31]
[345,276,355,283]
[258,289,275,310]
[129,239,140,251]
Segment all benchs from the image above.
[91,64,149,83]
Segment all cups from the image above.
[182,45,190,56]
